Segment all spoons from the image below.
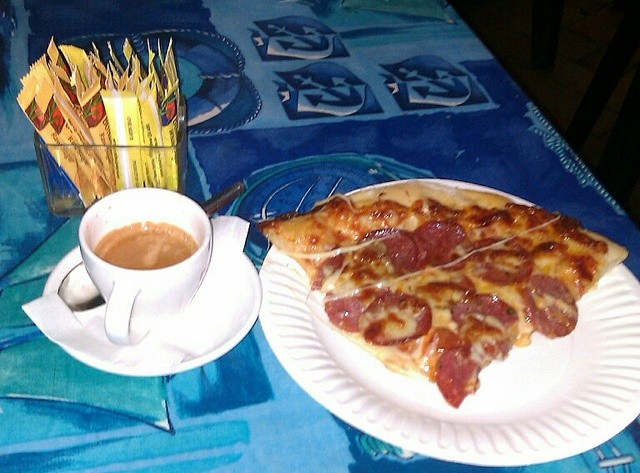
[57,177,246,312]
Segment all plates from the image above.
[259,179,639,467]
[43,229,261,375]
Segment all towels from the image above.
[20,215,251,379]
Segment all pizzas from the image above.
[262,179,629,407]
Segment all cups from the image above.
[79,187,212,344]
